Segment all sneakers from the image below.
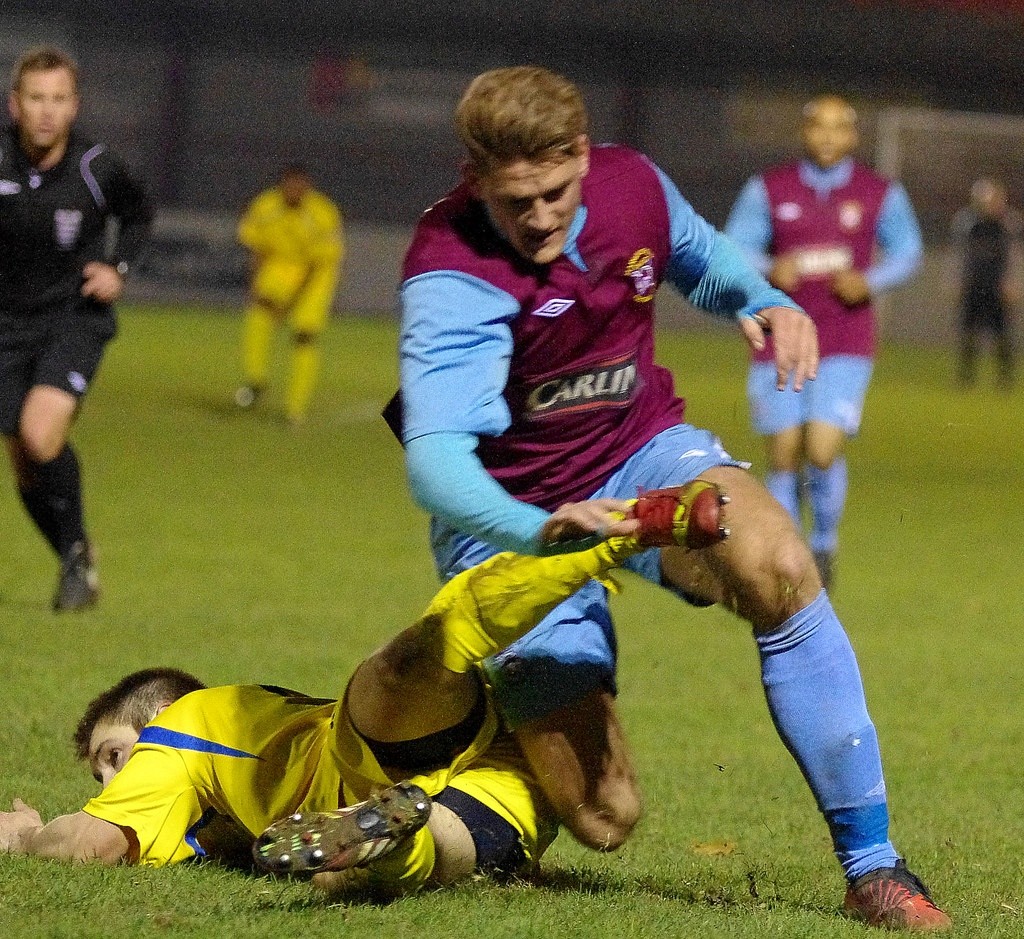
[844,858,952,931]
[53,562,100,614]
[253,779,432,876]
[631,479,730,550]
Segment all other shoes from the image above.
[813,547,834,588]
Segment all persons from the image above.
[381,67,950,929]
[0,481,731,897]
[230,163,344,422]
[724,91,930,587]
[949,175,1023,391]
[0,47,155,615]
